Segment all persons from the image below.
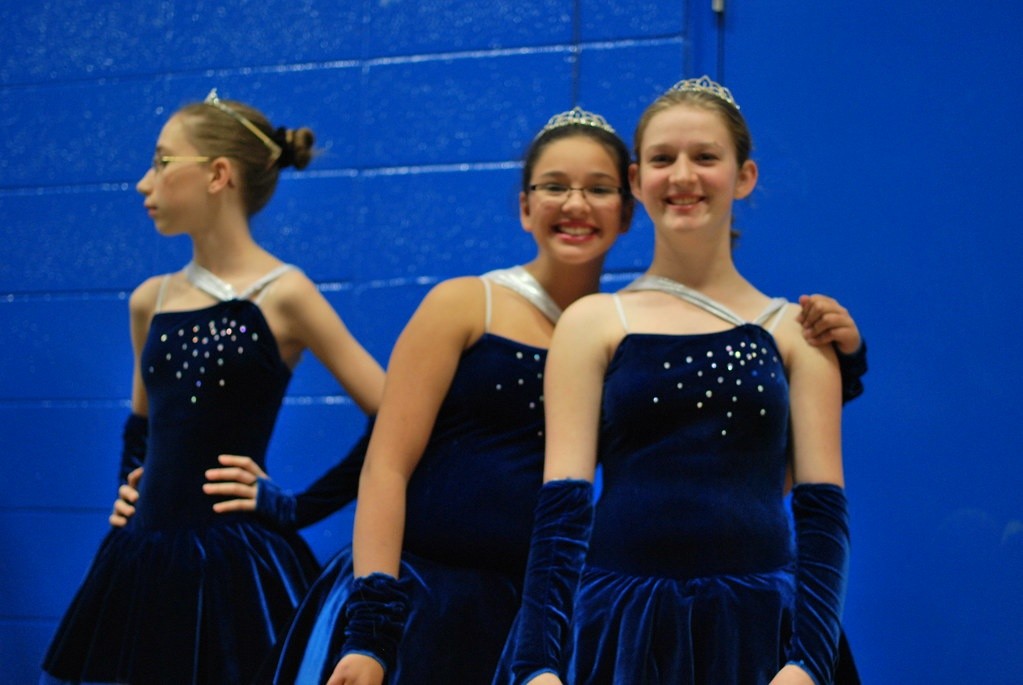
[37,90,384,685]
[274,106,866,685]
[490,76,862,685]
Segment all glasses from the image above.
[143,151,235,192]
[525,180,623,211]
